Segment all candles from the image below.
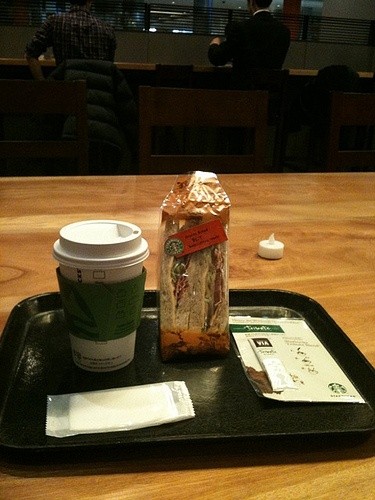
[258,233,284,260]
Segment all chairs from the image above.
[138,86,269,175]
[0,78,89,176]
[325,90,375,172]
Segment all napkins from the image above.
[68,383,179,432]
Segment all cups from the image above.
[52,219,150,372]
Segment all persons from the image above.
[25,0,119,80]
[207,0,290,89]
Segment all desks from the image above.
[0,171,375,500]
[0,58,375,174]
